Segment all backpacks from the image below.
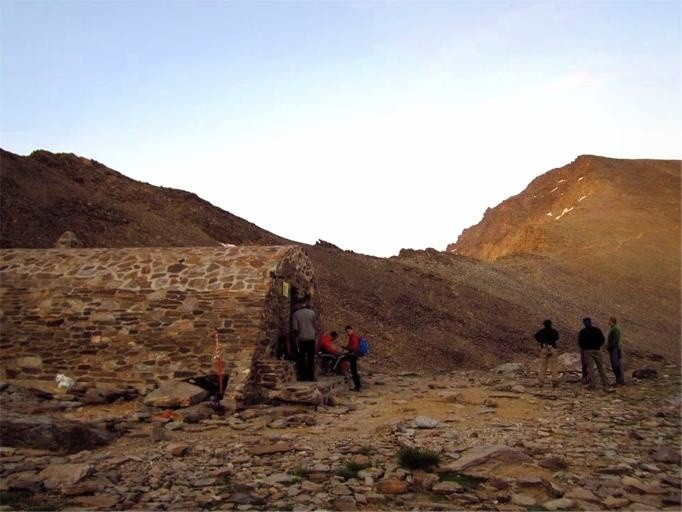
[358,336,369,354]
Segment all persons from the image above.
[605,316,624,384]
[344,324,368,391]
[577,316,610,388]
[534,319,561,387]
[320,330,349,375]
[291,296,318,381]
